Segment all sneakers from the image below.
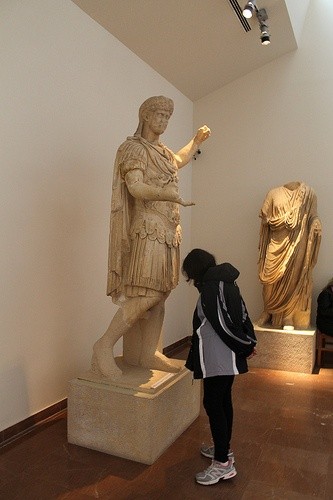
[201,447,236,464]
[195,460,237,485]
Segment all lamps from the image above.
[260,25,270,45]
[243,2,254,19]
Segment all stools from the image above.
[317,332,333,366]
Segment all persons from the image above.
[92,96,211,378]
[257,182,322,330]
[180,248,258,485]
